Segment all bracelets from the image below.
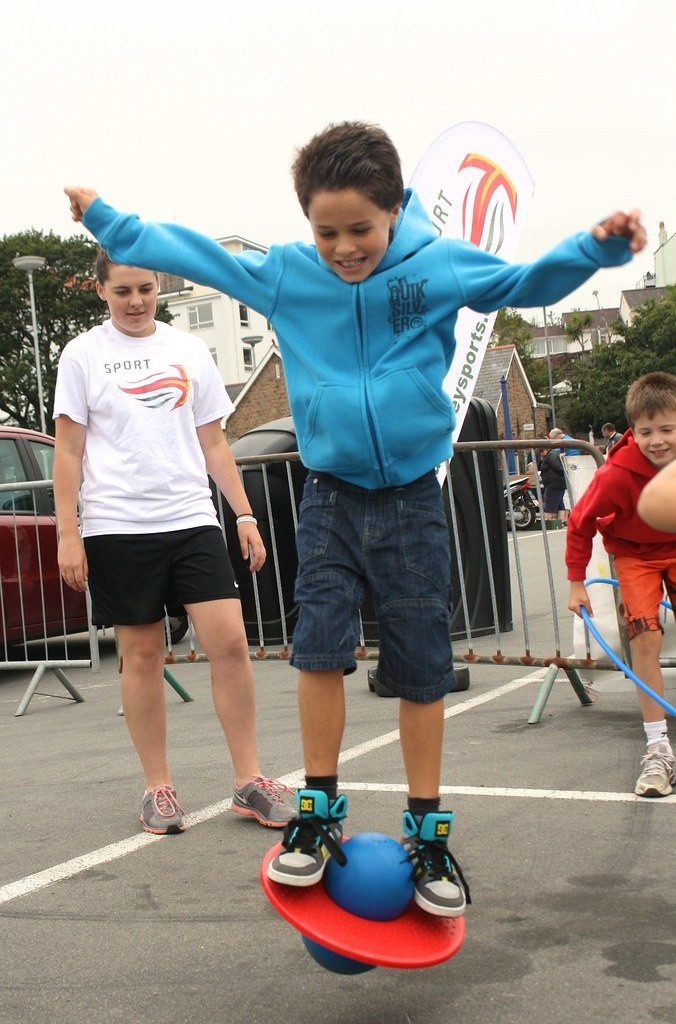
[236,512,259,525]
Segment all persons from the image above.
[526,423,581,520]
[50,244,302,833]
[636,456,676,535]
[563,369,676,798]
[60,116,652,921]
[600,421,626,456]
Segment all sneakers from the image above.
[399,809,472,917]
[266,788,348,887]
[635,742,676,796]
[140,785,186,834]
[231,775,299,827]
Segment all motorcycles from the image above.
[503,475,540,530]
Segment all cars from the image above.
[0,424,188,651]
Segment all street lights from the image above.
[13,255,50,436]
